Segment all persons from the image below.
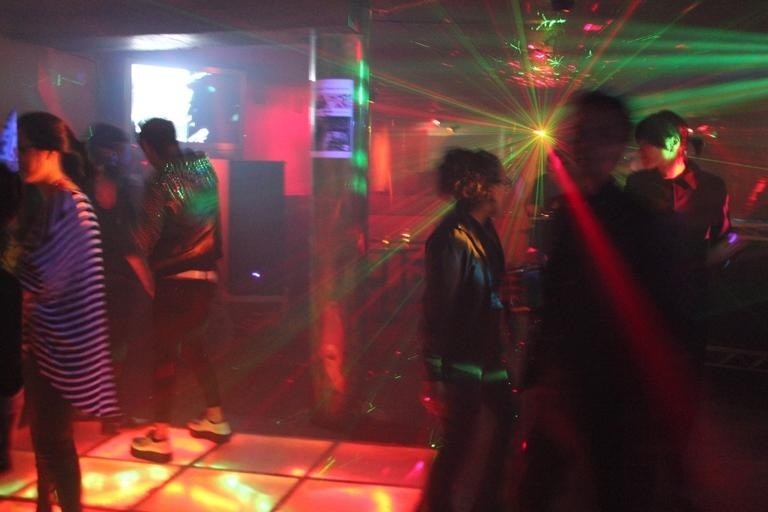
[511,91,645,512]
[419,147,517,512]
[2,112,119,512]
[81,121,154,433]
[0,158,24,475]
[128,118,233,465]
[627,109,743,512]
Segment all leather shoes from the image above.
[187,418,231,444]
[130,430,172,464]
